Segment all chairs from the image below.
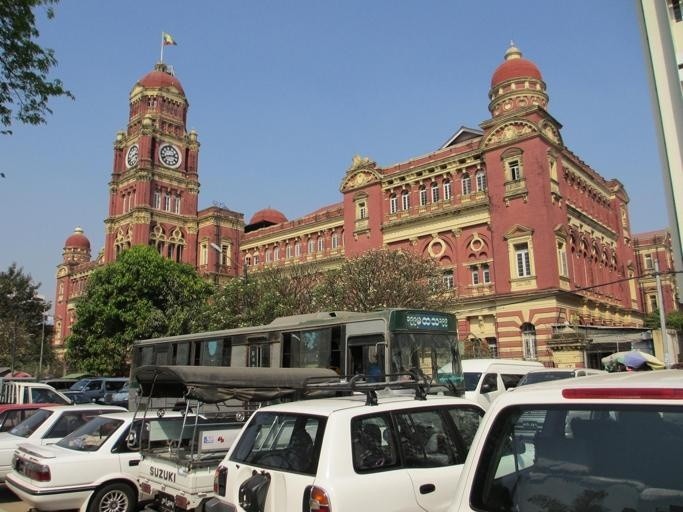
[364,423,382,448]
[289,429,313,460]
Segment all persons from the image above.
[622,352,653,371]
[365,363,398,382]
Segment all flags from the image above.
[162,32,177,46]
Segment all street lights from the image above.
[32,292,47,379]
[209,240,248,294]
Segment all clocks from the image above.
[125,144,138,168]
[158,144,183,169]
